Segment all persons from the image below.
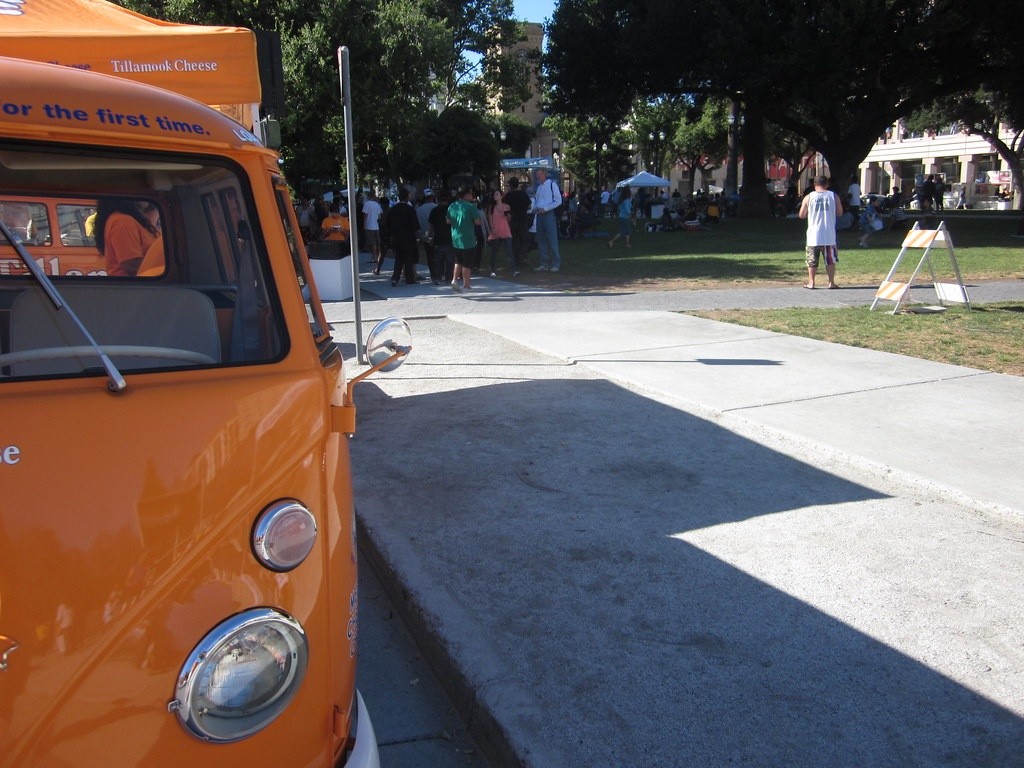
[918,174,945,212]
[956,187,967,209]
[559,191,597,240]
[83,197,161,277]
[294,177,535,291]
[601,188,726,232]
[608,187,633,248]
[845,175,907,245]
[799,176,843,289]
[531,168,562,271]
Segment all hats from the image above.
[507,176,518,184]
[423,189,434,196]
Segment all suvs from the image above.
[0,53,412,768]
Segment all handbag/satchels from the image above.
[551,181,564,216]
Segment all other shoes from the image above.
[534,265,549,271]
[392,280,398,287]
[372,268,380,275]
[804,285,813,289]
[451,281,460,291]
[860,242,868,248]
[551,267,559,272]
[608,241,612,247]
[829,285,840,289]
[513,271,520,277]
[490,272,501,278]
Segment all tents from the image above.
[616,170,671,193]
[0,0,262,139]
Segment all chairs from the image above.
[572,212,599,238]
[10,284,222,377]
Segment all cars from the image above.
[859,195,888,208]
